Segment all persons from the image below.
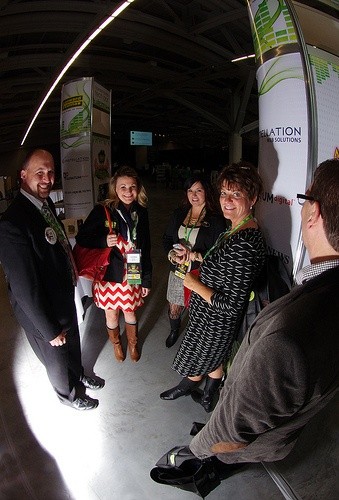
[0,146,106,412]
[159,162,269,414]
[163,173,229,349]
[72,164,154,365]
[188,159,339,481]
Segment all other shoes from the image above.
[80,375,103,391]
[60,394,95,411]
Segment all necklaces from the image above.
[184,213,205,226]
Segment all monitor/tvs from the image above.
[130,130,153,146]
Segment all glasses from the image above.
[296,193,310,206]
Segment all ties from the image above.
[41,202,79,287]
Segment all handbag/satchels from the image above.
[155,446,197,469]
[150,456,222,500]
[73,204,114,283]
[184,269,200,307]
[189,422,206,436]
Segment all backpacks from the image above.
[237,254,292,344]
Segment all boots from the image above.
[105,324,125,362]
[160,376,204,400]
[191,373,222,413]
[165,309,182,348]
[124,321,139,362]
